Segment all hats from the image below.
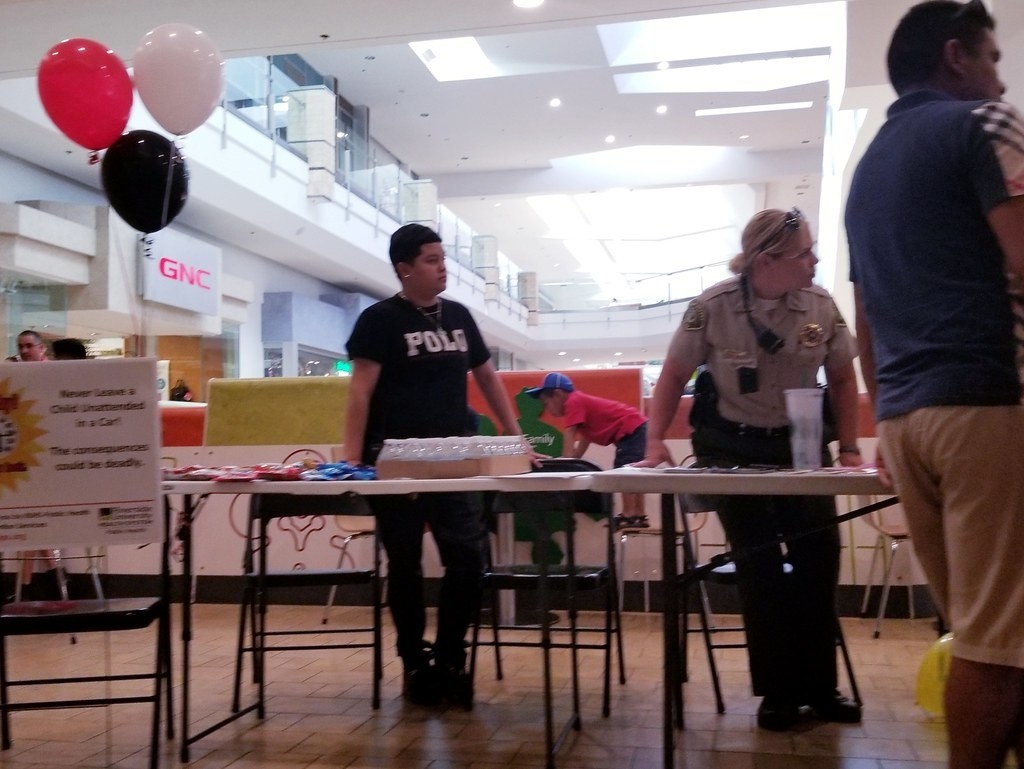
[525,372,574,397]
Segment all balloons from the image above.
[38,38,132,164]
[102,131,187,235]
[134,23,223,135]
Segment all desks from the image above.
[590,464,900,769]
[160,475,589,769]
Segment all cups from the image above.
[783,387,824,471]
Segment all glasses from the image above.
[943,0,989,24]
[766,208,802,244]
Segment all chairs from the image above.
[676,461,866,716]
[229,493,387,721]
[859,495,917,641]
[470,455,627,721]
[320,447,387,625]
[0,496,176,769]
[619,512,714,628]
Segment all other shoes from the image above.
[758,695,800,733]
[807,689,862,723]
[435,664,473,709]
[401,663,448,710]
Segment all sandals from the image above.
[604,512,649,528]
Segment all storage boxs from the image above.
[375,453,531,480]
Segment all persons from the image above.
[634,206,862,729]
[346,222,551,712]
[525,373,651,530]
[18,330,49,362]
[846,1,1024,769]
[52,338,87,360]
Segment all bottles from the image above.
[376,435,531,478]
